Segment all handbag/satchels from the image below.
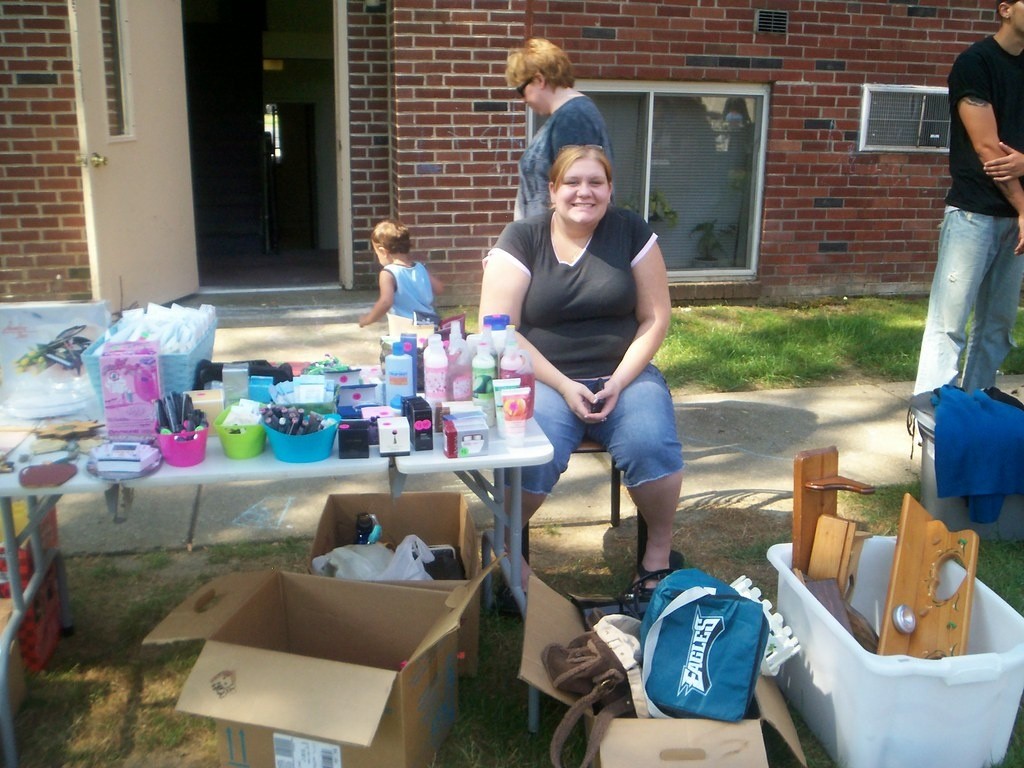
[540,613,652,768]
[639,570,770,722]
[311,534,435,581]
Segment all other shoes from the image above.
[982,386,1024,410]
[495,574,522,611]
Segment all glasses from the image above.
[516,77,535,97]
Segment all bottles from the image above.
[354,511,398,555]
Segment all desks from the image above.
[0,363,554,768]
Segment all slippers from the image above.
[631,548,685,600]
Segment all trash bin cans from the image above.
[908,388,1024,541]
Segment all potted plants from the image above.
[688,218,736,267]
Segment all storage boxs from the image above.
[308,496,482,677]
[521,574,808,767]
[767,536,1024,768]
[0,506,62,673]
[143,553,506,767]
[81,317,490,458]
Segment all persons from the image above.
[669,93,751,270]
[478,145,684,615]
[505,37,614,222]
[914,0,1024,395]
[359,219,443,331]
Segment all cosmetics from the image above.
[94,341,162,472]
[154,390,209,441]
[325,312,535,459]
[184,363,334,437]
